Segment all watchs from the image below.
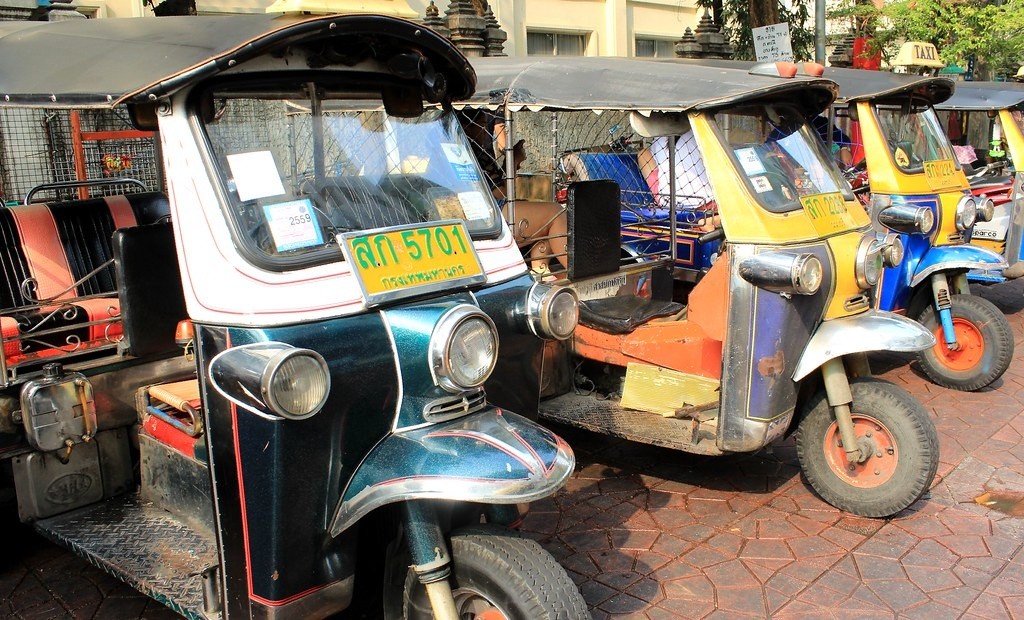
[840,147,851,152]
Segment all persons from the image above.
[649,129,721,233]
[457,108,568,282]
[765,116,854,170]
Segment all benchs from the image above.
[0,180,171,378]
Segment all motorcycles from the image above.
[718,58,1024,394]
[456,47,940,520]
[1,13,596,620]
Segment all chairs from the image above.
[566,180,686,367]
[115,215,214,465]
